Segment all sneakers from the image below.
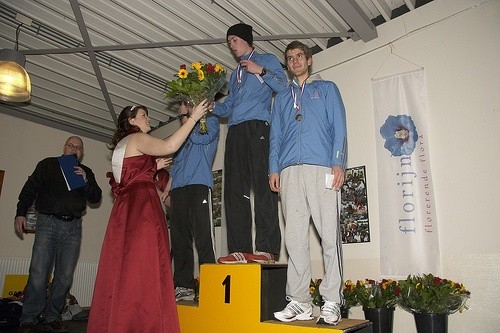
[248,250,274,264]
[316,300,341,325]
[41,319,69,332]
[20,320,34,333]
[272,296,313,322]
[174,287,195,302]
[218,252,250,264]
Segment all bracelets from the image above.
[190,117,197,122]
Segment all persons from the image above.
[160,100,219,300]
[268,41,347,325]
[14,136,102,333]
[86,97,208,333]
[207,23,281,265]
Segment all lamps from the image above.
[0,23,32,104]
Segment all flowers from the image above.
[355,279,402,307]
[393,273,470,314]
[163,61,227,135]
[304,279,357,306]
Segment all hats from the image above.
[227,24,253,45]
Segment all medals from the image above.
[232,86,240,94]
[295,113,303,122]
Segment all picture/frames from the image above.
[340,166,370,244]
[211,169,222,228]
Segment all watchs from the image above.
[180,114,188,119]
[259,68,266,77]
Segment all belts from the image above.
[39,211,80,221]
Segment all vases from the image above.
[320,301,350,319]
[414,312,450,332]
[362,304,396,333]
